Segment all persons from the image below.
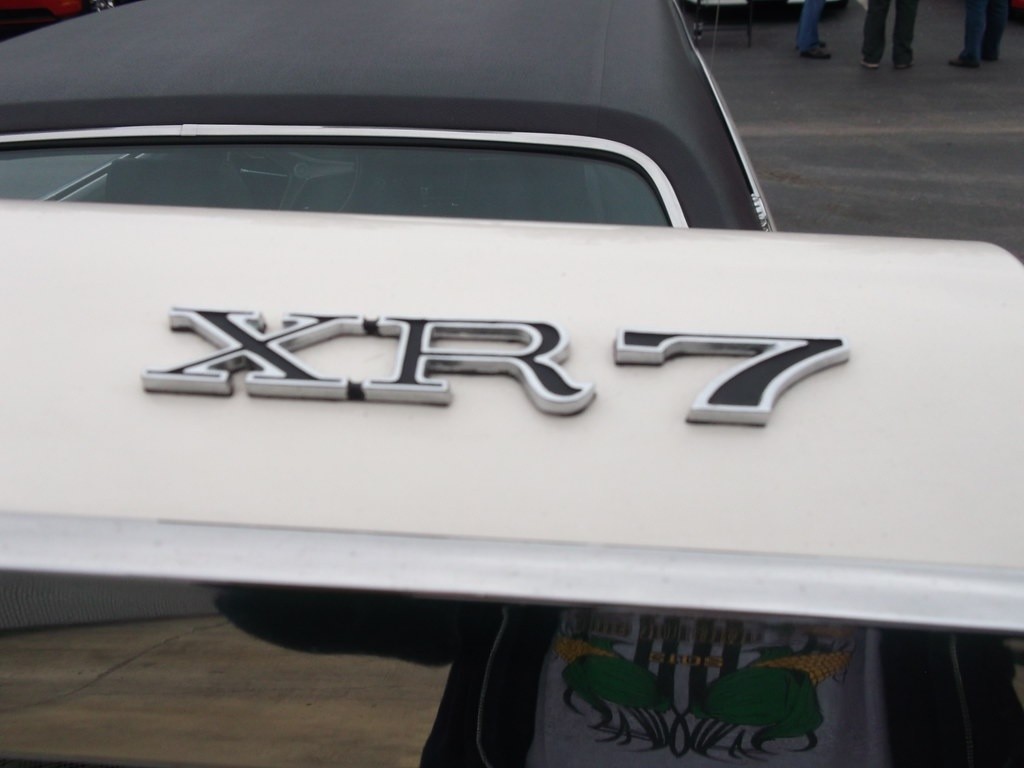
[201,581,1024,768]
[860,0,918,70]
[947,0,1009,69]
[793,0,834,60]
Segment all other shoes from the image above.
[980,50,998,60]
[801,48,830,59]
[895,56,915,68]
[797,40,825,49]
[949,55,981,68]
[861,57,879,68]
